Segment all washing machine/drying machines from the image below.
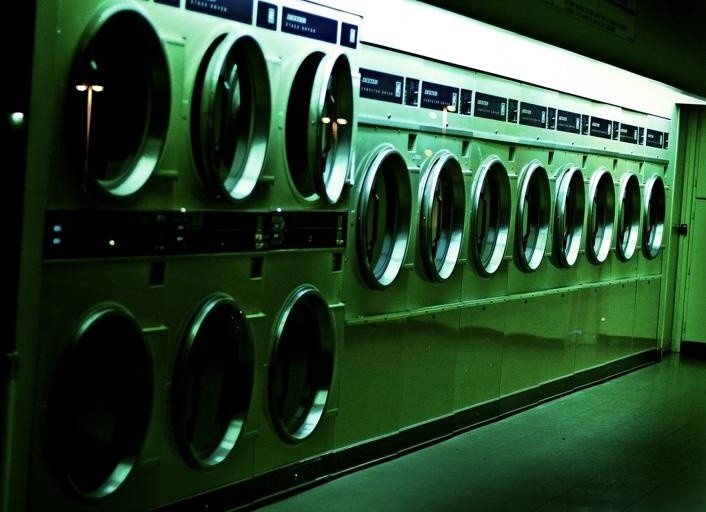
[2,0,367,510]
[334,38,671,480]
